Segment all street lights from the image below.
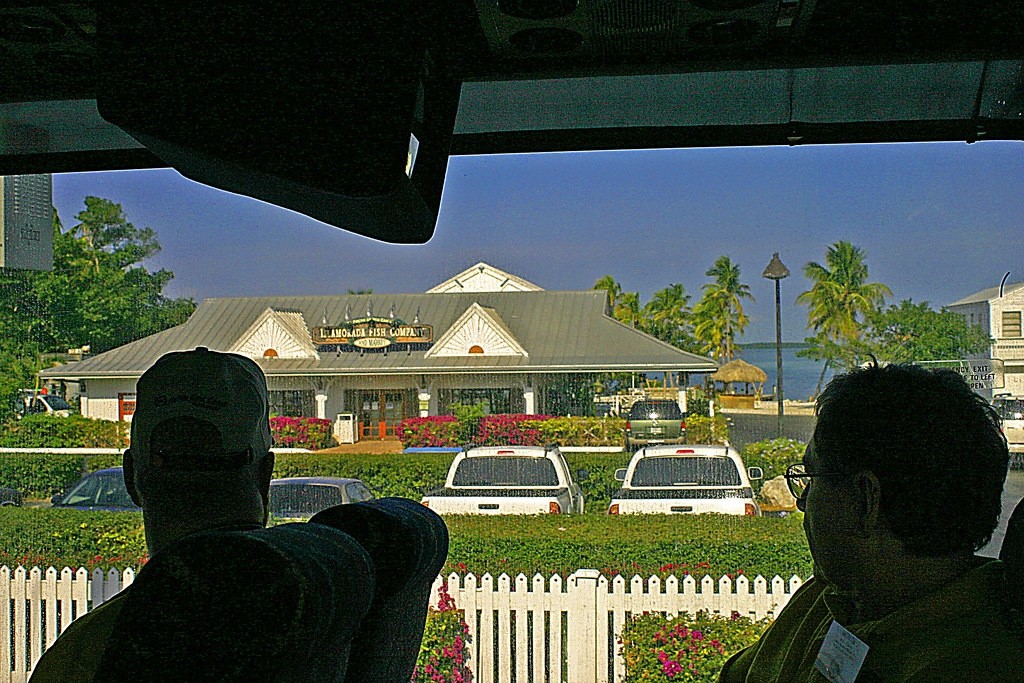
[762,252,790,417]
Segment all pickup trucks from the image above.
[418,446,588,520]
[603,442,765,518]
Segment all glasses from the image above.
[782,462,868,501]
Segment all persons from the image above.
[25,346,450,683]
[60,379,67,401]
[51,384,57,395]
[718,363,1024,683]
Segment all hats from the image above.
[130,347,272,470]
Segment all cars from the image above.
[0,485,25,511]
[51,465,144,515]
[269,478,375,523]
[19,390,74,417]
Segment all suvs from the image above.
[625,398,687,452]
[987,393,1024,465]
